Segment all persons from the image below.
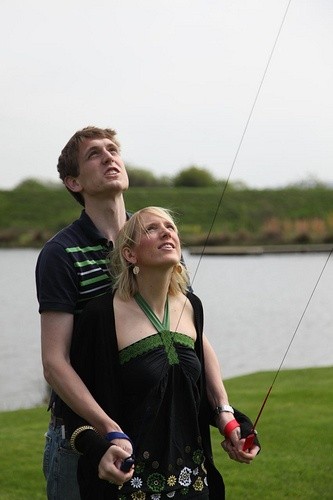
[60,206,262,500]
[34,127,242,500]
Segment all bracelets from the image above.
[69,425,99,455]
[217,406,234,415]
